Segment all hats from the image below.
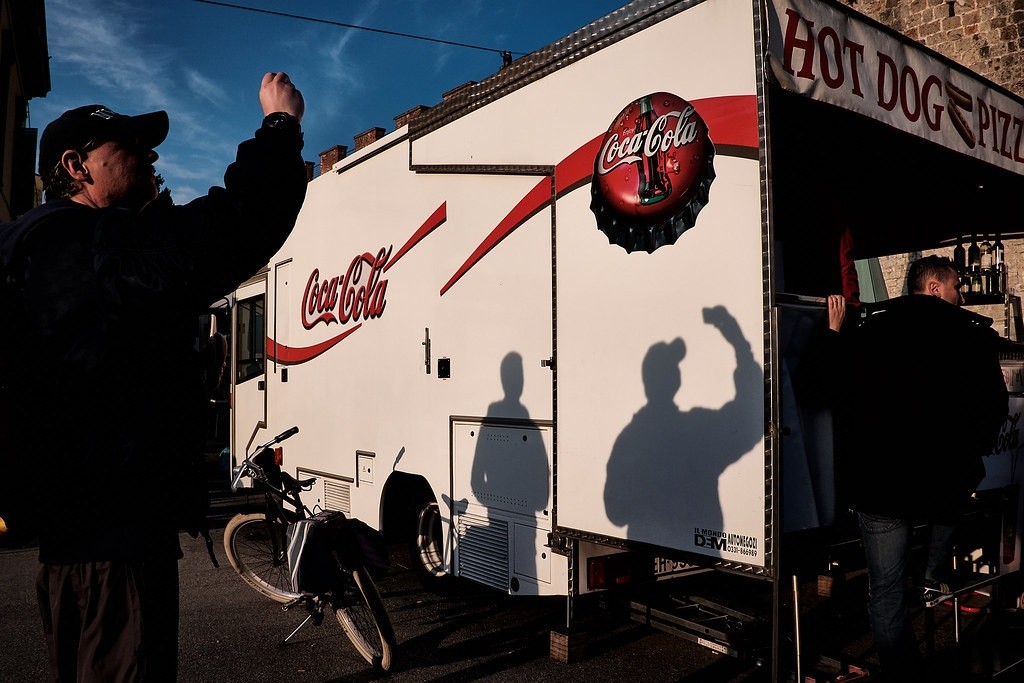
[40,104,170,177]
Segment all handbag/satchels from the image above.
[344,518,410,583]
[286,518,338,593]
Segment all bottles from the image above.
[634,96,672,205]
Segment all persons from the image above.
[814,255,1010,683]
[0,70,307,683]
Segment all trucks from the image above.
[198,2,1023,683]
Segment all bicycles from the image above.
[222,426,399,674]
